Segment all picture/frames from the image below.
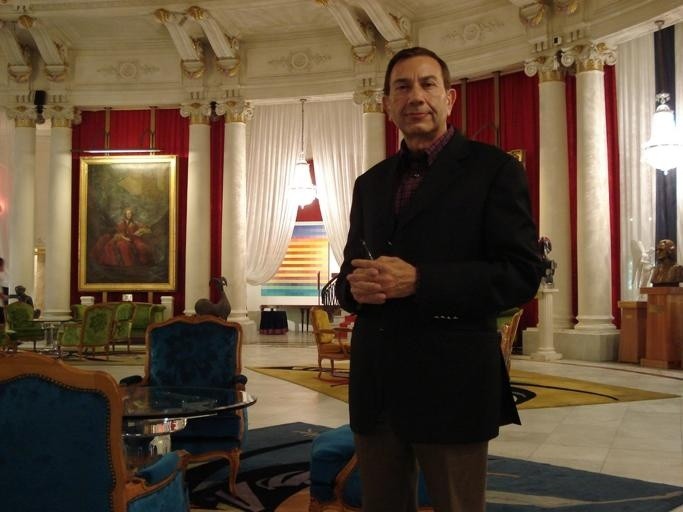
[78,155,180,291]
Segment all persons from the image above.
[8,286,34,312]
[537,236,557,285]
[114,209,152,267]
[337,45,544,511]
[1,260,9,332]
[652,238,683,285]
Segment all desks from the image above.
[260,311,288,335]
[23,316,73,352]
[113,384,257,468]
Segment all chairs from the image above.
[499,308,525,377]
[4,301,45,352]
[57,303,111,357]
[0,332,9,352]
[308,305,352,377]
[111,302,136,352]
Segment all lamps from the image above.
[643,19,683,177]
[286,98,316,210]
[35,105,47,125]
[210,101,220,122]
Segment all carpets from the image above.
[246,362,683,414]
[59,345,150,367]
[184,421,683,512]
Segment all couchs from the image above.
[118,314,248,502]
[70,302,166,352]
[273,424,435,512]
[0,353,190,512]
